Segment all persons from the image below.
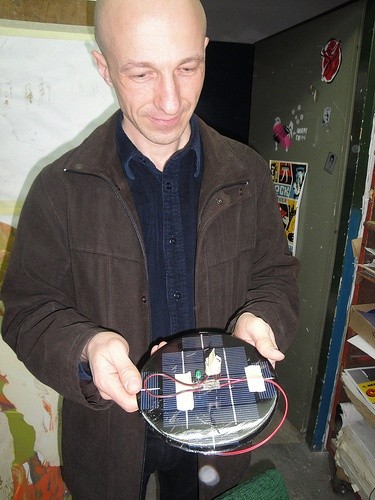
[0,0,301,500]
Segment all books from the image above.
[332,246,375,500]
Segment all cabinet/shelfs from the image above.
[328,162,375,500]
